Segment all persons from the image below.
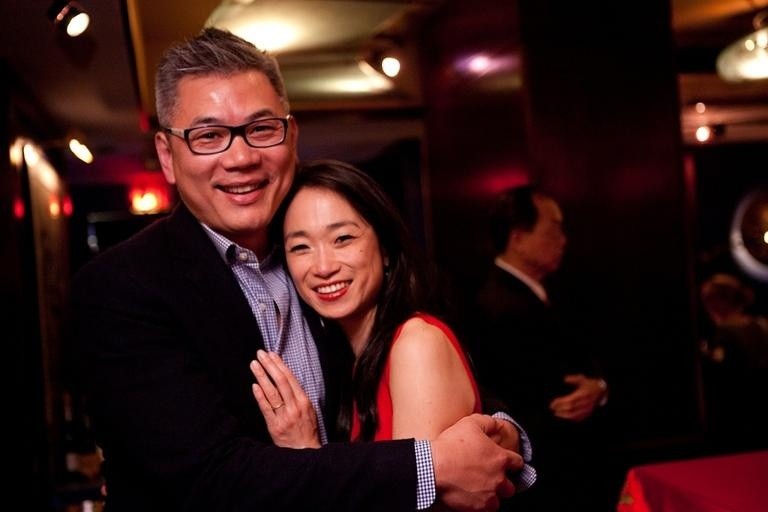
[696,266,766,453]
[60,25,543,512]
[474,182,616,431]
[251,156,482,447]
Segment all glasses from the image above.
[160,113,293,157]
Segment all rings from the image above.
[272,401,285,411]
